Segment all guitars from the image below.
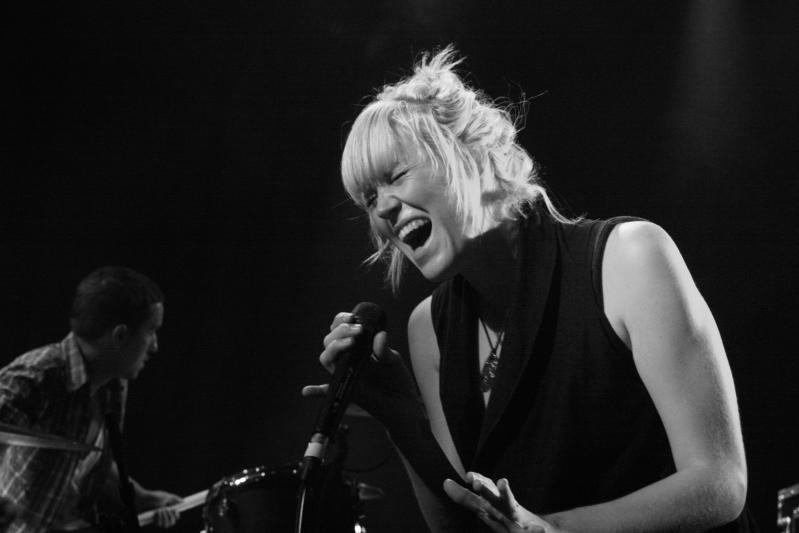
[66,465,269,533]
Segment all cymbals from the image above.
[0,421,102,453]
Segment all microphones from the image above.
[296,303,385,487]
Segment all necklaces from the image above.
[477,310,510,393]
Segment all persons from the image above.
[0,266,184,533]
[301,45,748,533]
